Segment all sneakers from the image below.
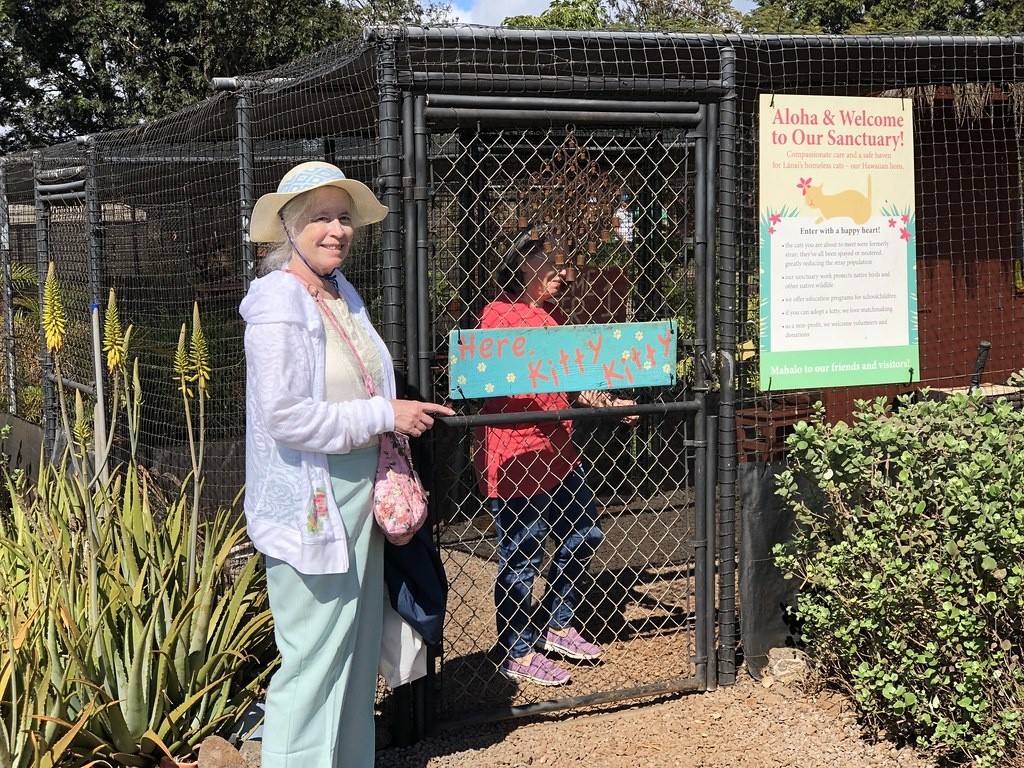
[499,652,571,690]
[533,626,603,662]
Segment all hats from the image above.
[249,160,390,245]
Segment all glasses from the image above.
[534,253,574,270]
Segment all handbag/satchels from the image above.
[371,429,429,551]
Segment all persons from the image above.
[477,227,643,685]
[239,160,459,768]
